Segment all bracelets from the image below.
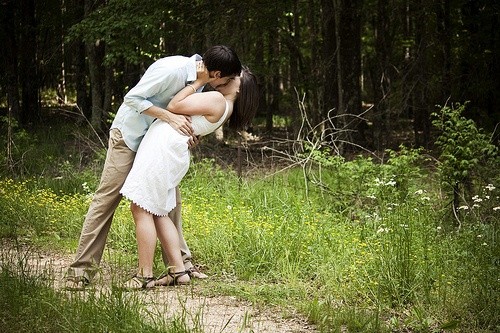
[186,84,197,93]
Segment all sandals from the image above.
[153,269,191,286]
[114,274,156,292]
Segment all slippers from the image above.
[65,276,86,291]
[185,265,208,279]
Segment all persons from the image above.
[64,46,242,292]
[120,60,259,290]
